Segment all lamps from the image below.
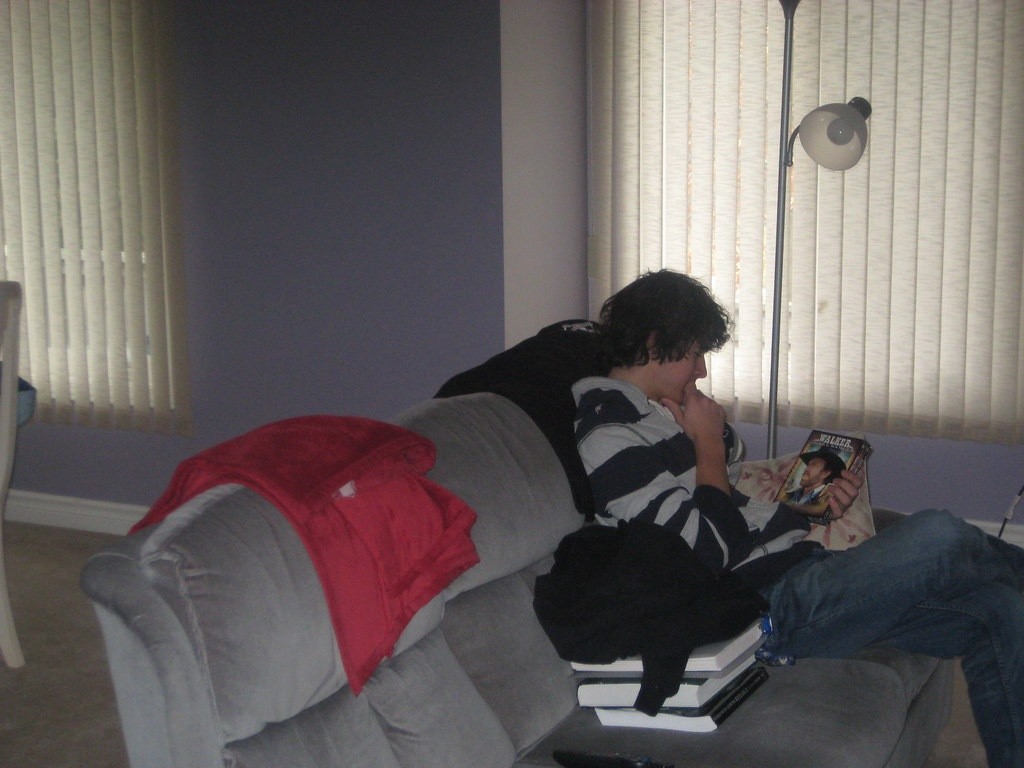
[768,0,874,460]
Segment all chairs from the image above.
[0,282,27,668]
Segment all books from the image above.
[567,618,770,732]
[773,430,872,526]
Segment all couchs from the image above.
[79,392,953,768]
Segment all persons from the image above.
[572,270,1024,768]
[787,443,846,506]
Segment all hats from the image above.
[799,443,846,483]
